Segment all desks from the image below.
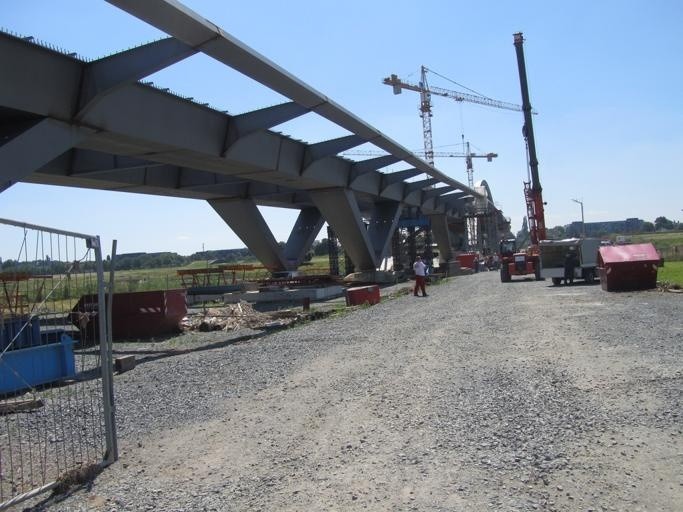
[599,264,656,291]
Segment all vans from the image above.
[269,269,306,277]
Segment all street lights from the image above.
[569,197,586,233]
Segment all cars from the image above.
[600,238,614,246]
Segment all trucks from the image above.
[536,234,601,287]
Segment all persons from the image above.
[473,253,499,273]
[413,256,429,296]
[563,247,576,285]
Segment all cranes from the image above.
[381,63,539,192]
[341,132,500,191]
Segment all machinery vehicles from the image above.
[497,31,548,285]
